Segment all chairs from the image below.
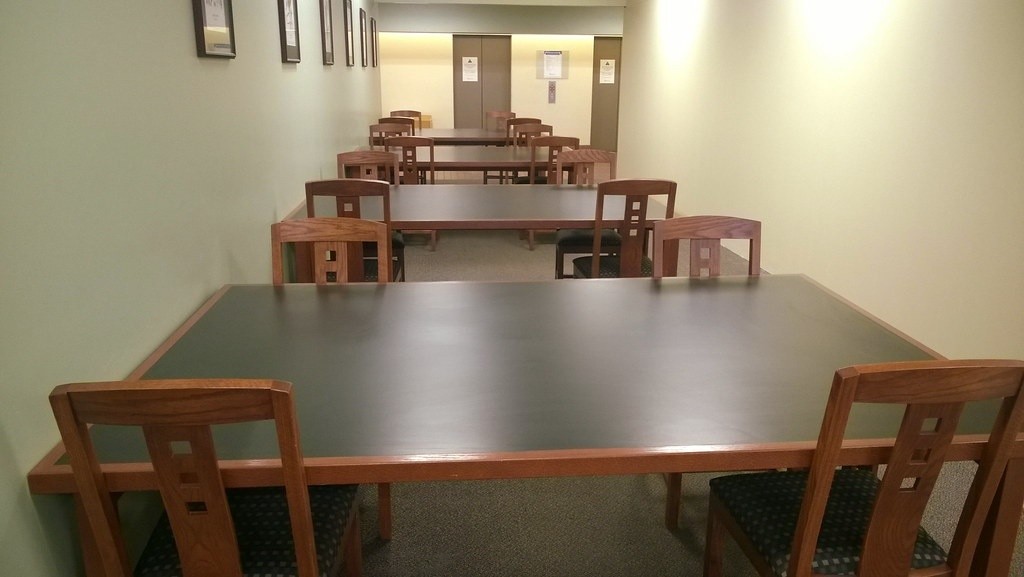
[556,150,621,280]
[270,216,393,541]
[651,216,761,527]
[49,378,362,577]
[706,359,1023,577]
[337,111,580,250]
[572,180,678,279]
[337,153,406,282]
[304,178,403,284]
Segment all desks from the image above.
[347,145,579,184]
[280,184,668,229]
[28,273,1024,494]
[369,125,546,147]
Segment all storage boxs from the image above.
[413,114,432,127]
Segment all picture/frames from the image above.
[344,0,354,66]
[320,0,334,65]
[193,0,237,59]
[359,8,368,66]
[372,18,377,67]
[278,0,302,62]
